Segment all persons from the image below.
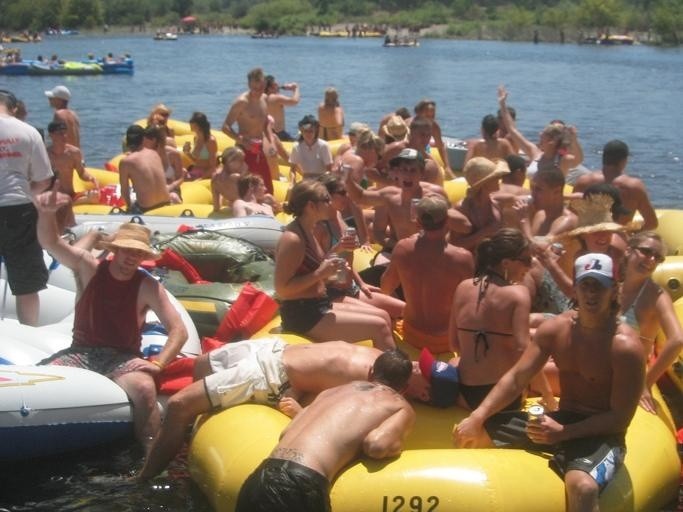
[533,26,612,44]
[253,18,418,48]
[233,349,416,512]
[1,67,683,481]
[452,252,646,512]
[0,22,131,64]
[165,20,207,35]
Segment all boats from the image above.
[586,35,632,44]
[2,35,42,43]
[385,42,420,47]
[0,56,134,74]
[319,31,381,37]
[154,32,177,38]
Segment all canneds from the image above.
[345,227,356,252]
[527,404,545,439]
[337,257,346,284]
[525,194,535,205]
[340,164,351,181]
[410,198,421,222]
[551,242,563,255]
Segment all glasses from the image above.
[330,188,346,196]
[630,245,664,264]
[316,193,333,206]
[510,255,532,267]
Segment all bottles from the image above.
[277,83,293,91]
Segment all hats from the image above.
[382,115,409,141]
[551,183,645,242]
[416,191,447,226]
[388,149,425,177]
[418,347,460,408]
[414,100,435,113]
[573,253,619,287]
[344,122,368,135]
[462,155,531,191]
[297,115,317,131]
[98,222,160,261]
[43,85,70,101]
